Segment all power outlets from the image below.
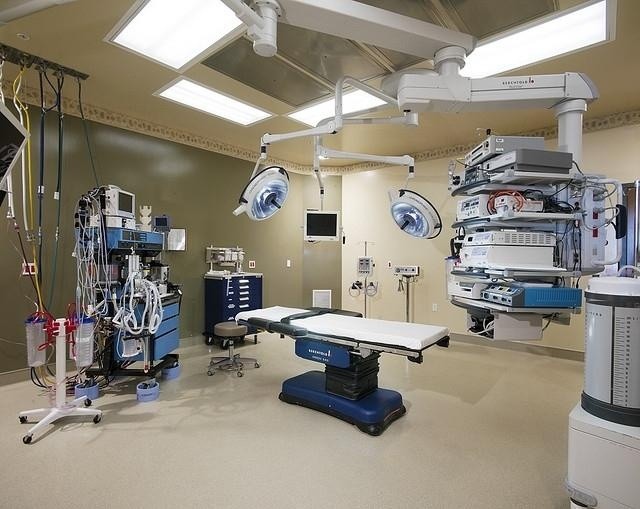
[367,281,377,290]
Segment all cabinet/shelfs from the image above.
[84,226,181,382]
[205,274,262,348]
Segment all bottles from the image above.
[74,383,99,399]
[74,318,95,366]
[25,317,46,367]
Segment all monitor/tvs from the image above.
[304,211,338,241]
[106,188,137,219]
[0,102,30,187]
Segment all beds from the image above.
[239,306,449,434]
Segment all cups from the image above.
[159,282,167,295]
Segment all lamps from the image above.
[232,75,397,221]
[316,113,443,240]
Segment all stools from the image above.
[208,322,260,376]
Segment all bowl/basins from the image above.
[161,364,181,380]
[136,382,159,402]
